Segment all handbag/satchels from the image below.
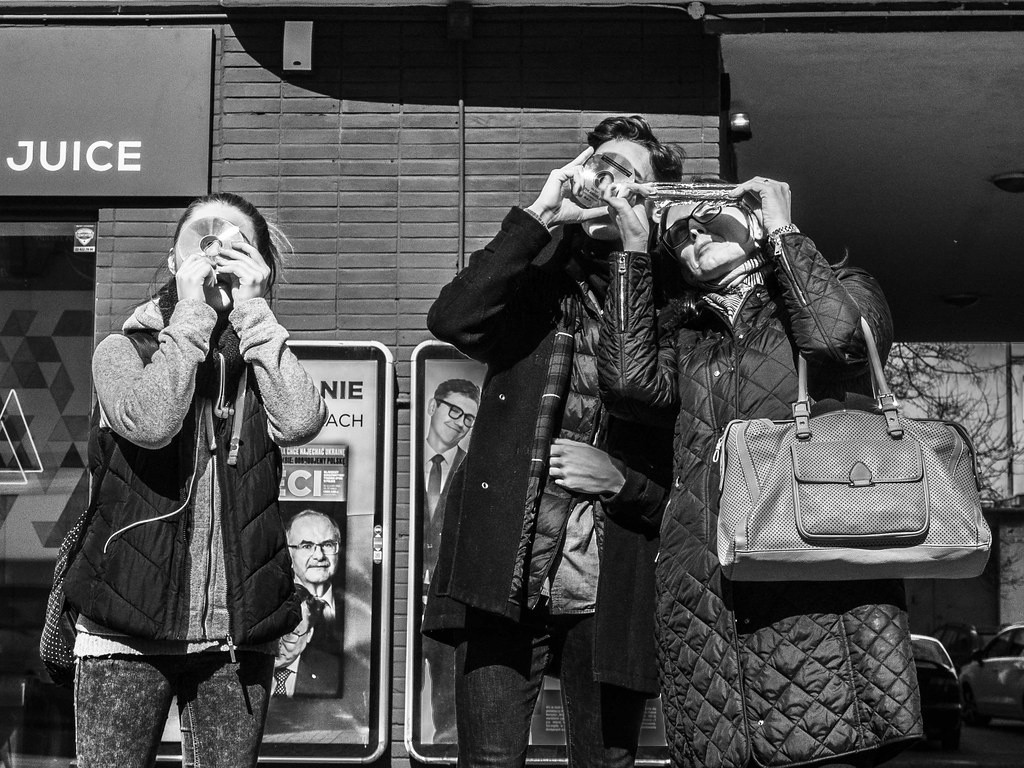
[38,507,89,690]
[713,316,993,583]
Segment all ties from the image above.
[427,453,445,522]
[314,601,327,628]
[273,667,296,697]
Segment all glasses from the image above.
[658,199,743,262]
[287,542,340,555]
[281,628,311,644]
[434,397,476,428]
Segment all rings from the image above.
[763,179,769,183]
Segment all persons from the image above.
[419,115,686,768]
[285,509,341,657]
[595,174,925,768]
[269,583,340,697]
[422,378,482,745]
[62,194,329,768]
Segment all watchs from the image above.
[768,223,801,246]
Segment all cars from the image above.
[907,632,965,753]
[956,623,1024,727]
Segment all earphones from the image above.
[214,406,228,419]
[221,407,234,416]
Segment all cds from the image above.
[177,217,244,275]
[571,153,636,208]
